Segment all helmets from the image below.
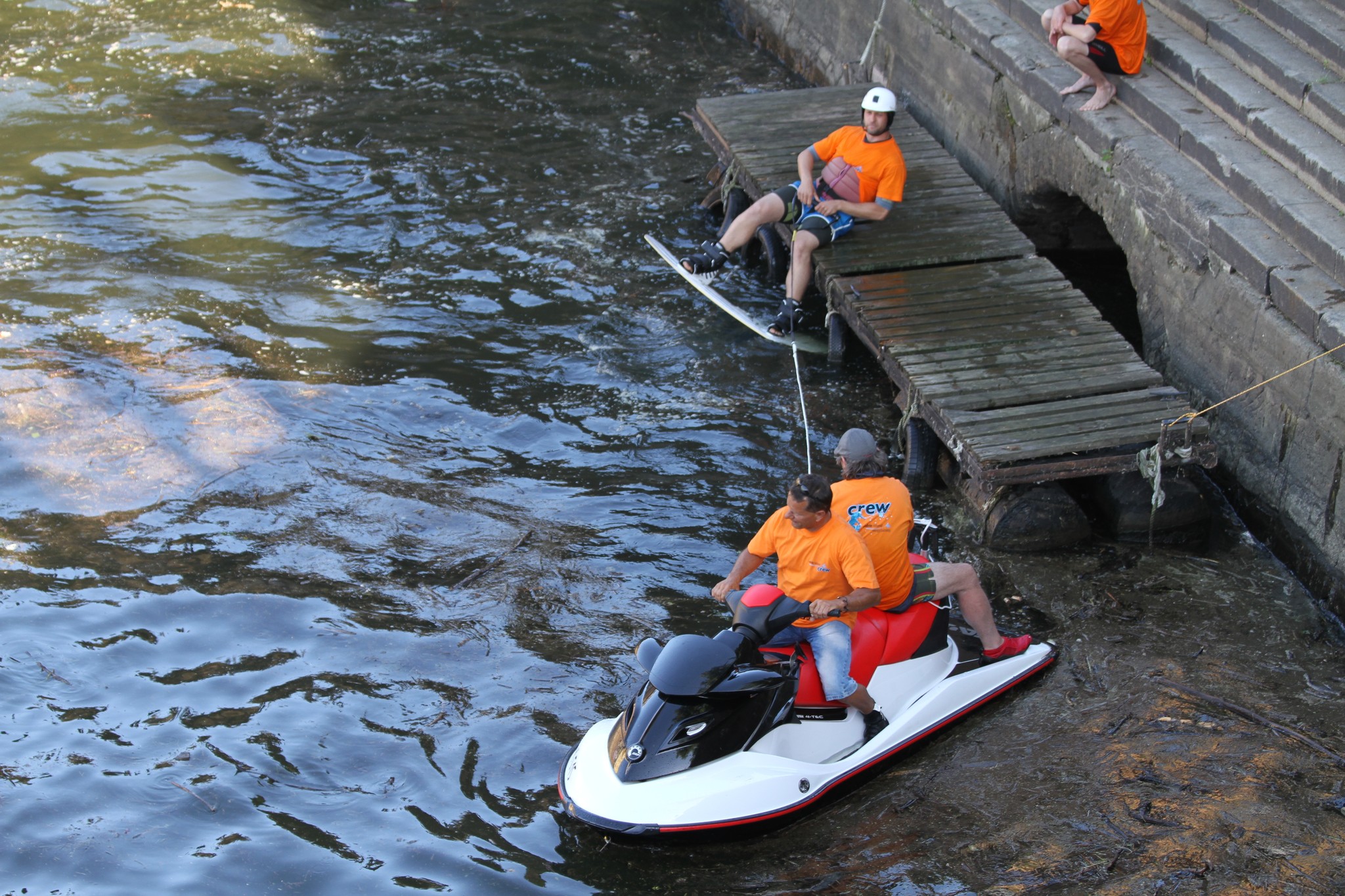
[861,86,896,112]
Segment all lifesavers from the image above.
[887,416,938,490]
[738,224,784,286]
[824,314,848,363]
[702,183,748,238]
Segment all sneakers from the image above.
[982,634,1032,662]
[860,698,890,747]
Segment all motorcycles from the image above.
[556,494,1060,839]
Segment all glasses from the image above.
[796,477,826,505]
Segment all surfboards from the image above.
[643,231,834,356]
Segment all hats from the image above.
[834,428,877,459]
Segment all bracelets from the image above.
[837,596,848,613]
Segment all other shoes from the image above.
[677,239,730,284]
[766,299,806,340]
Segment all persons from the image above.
[1041,0,1147,111]
[680,87,906,337]
[711,474,889,742]
[829,428,1033,664]
